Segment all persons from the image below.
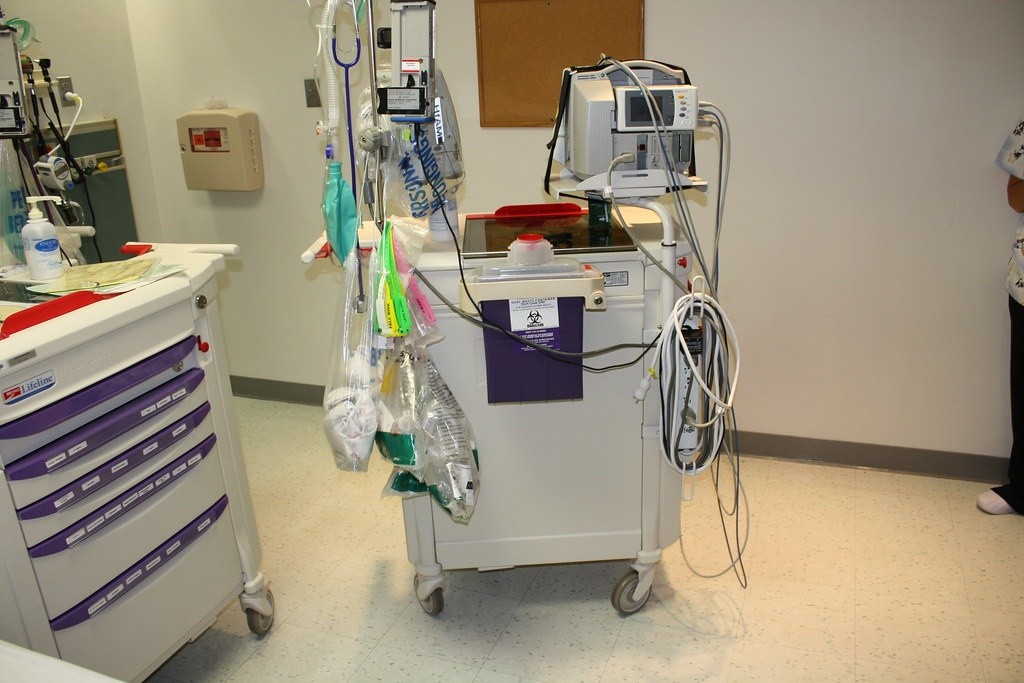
[973,120,1024,518]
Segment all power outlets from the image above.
[57,76,76,107]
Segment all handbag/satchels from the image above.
[319,249,480,526]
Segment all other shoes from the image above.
[975,490,1015,514]
